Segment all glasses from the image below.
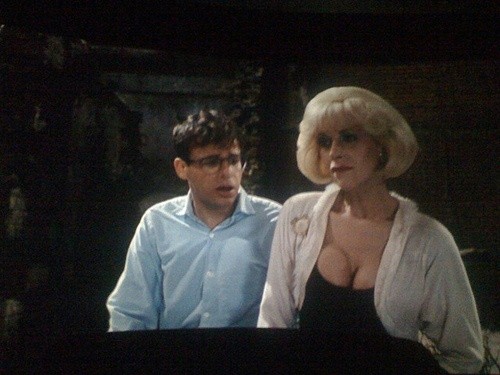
[316,132,373,148]
[184,153,248,173]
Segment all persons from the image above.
[256,86,485,375]
[102,106,284,332]
